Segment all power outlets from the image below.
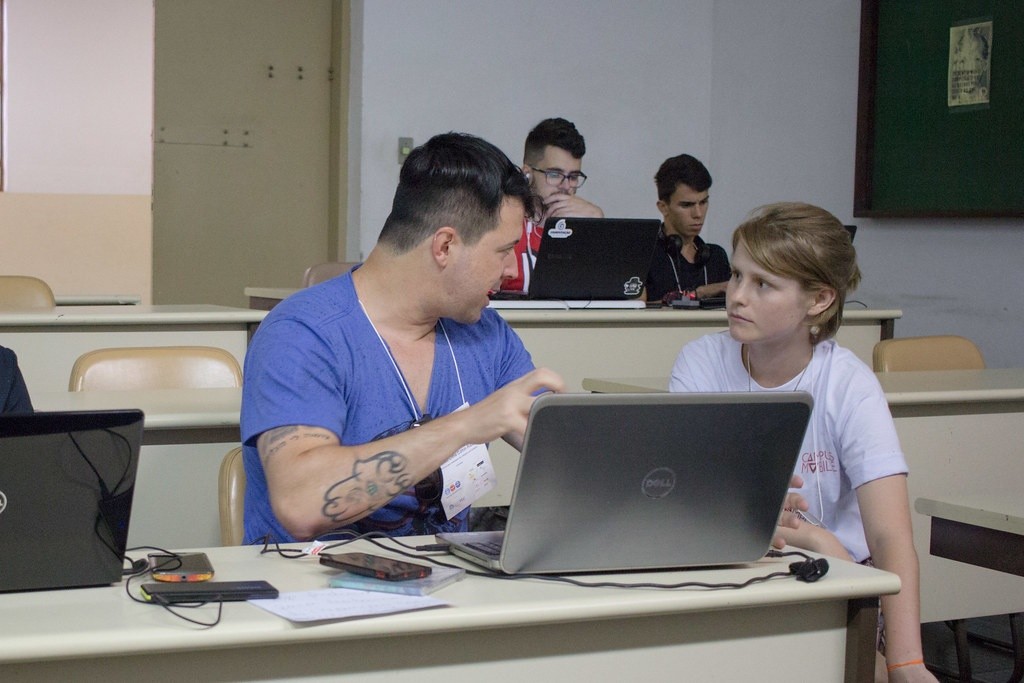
[399,137,414,164]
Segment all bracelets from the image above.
[694,289,697,300]
[887,659,924,671]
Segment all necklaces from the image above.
[748,352,812,392]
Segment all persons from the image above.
[239,133,567,546]
[0,345,34,413]
[950,33,989,105]
[669,202,940,683]
[644,154,731,304]
[488,117,604,294]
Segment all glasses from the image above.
[530,164,587,188]
[410,414,444,503]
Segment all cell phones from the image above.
[319,552,432,582]
[146,552,214,583]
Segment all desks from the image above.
[913,485,1024,577]
[26,384,245,553]
[0,303,269,393]
[582,367,1024,624]
[471,309,903,509]
[0,530,901,683]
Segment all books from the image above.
[327,561,466,596]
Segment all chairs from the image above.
[873,335,986,374]
[0,275,56,307]
[68,345,245,393]
[217,447,246,547]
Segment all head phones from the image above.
[659,222,711,268]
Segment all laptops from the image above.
[0,409,144,590]
[487,218,662,301]
[434,389,815,576]
